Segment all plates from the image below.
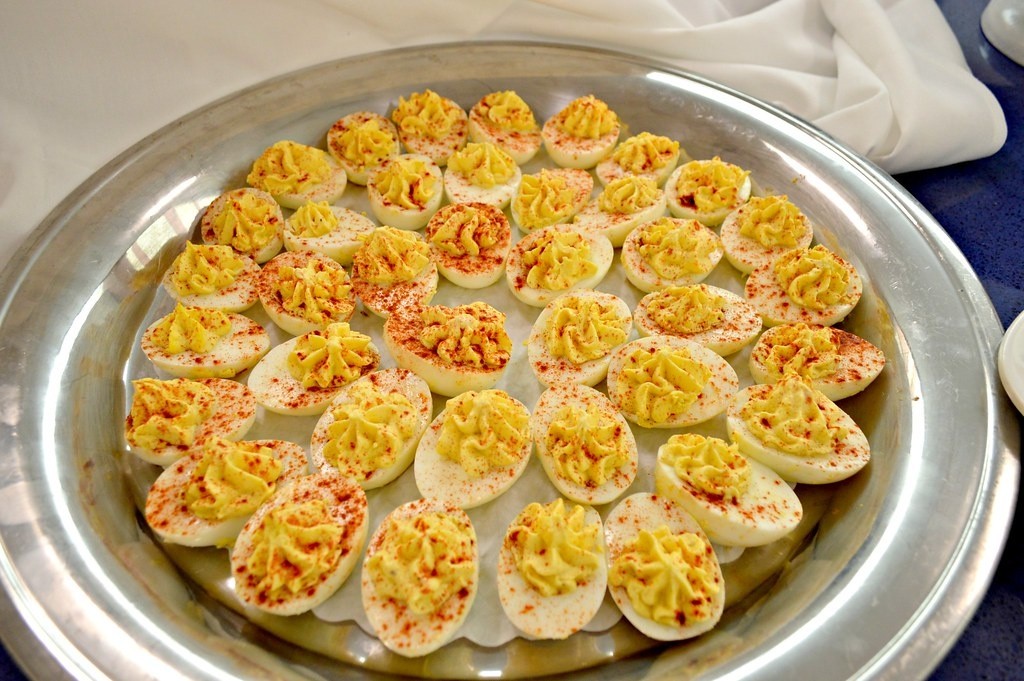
[997,309,1024,417]
[0,39,1020,681]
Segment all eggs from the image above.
[123,91,886,658]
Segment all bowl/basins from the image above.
[981,1,1024,67]
